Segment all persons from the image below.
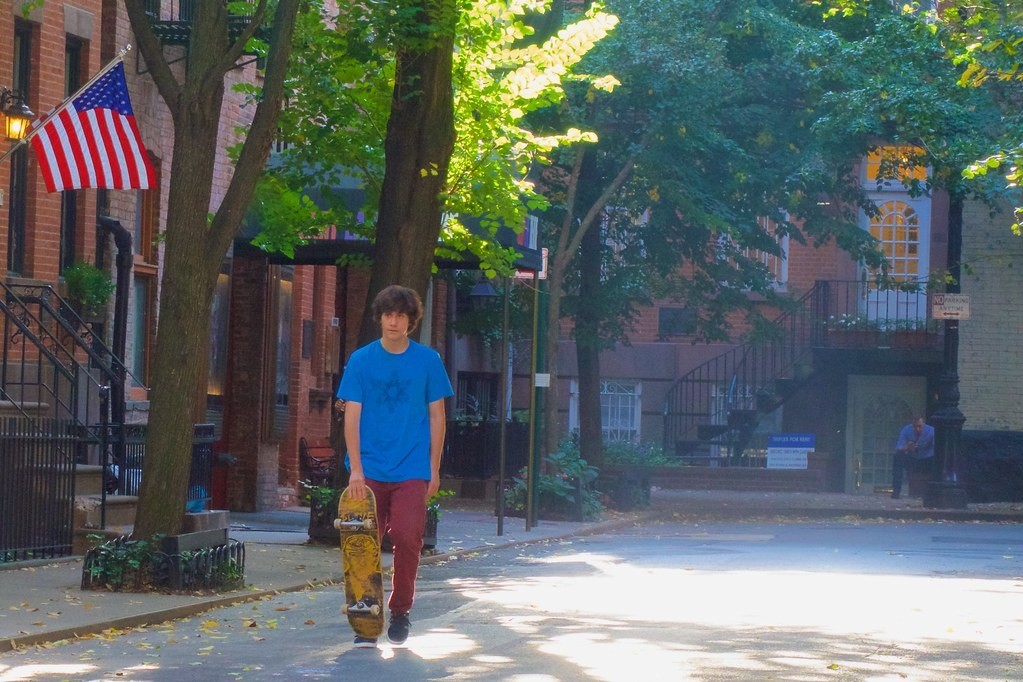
[335,284,453,648]
[892,414,934,507]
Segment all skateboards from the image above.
[332,483,384,639]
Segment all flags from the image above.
[25,60,156,193]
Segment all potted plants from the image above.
[62,262,114,323]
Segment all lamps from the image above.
[0,85,34,146]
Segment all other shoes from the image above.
[892,487,901,499]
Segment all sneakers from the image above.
[353,635,377,648]
[387,610,412,645]
